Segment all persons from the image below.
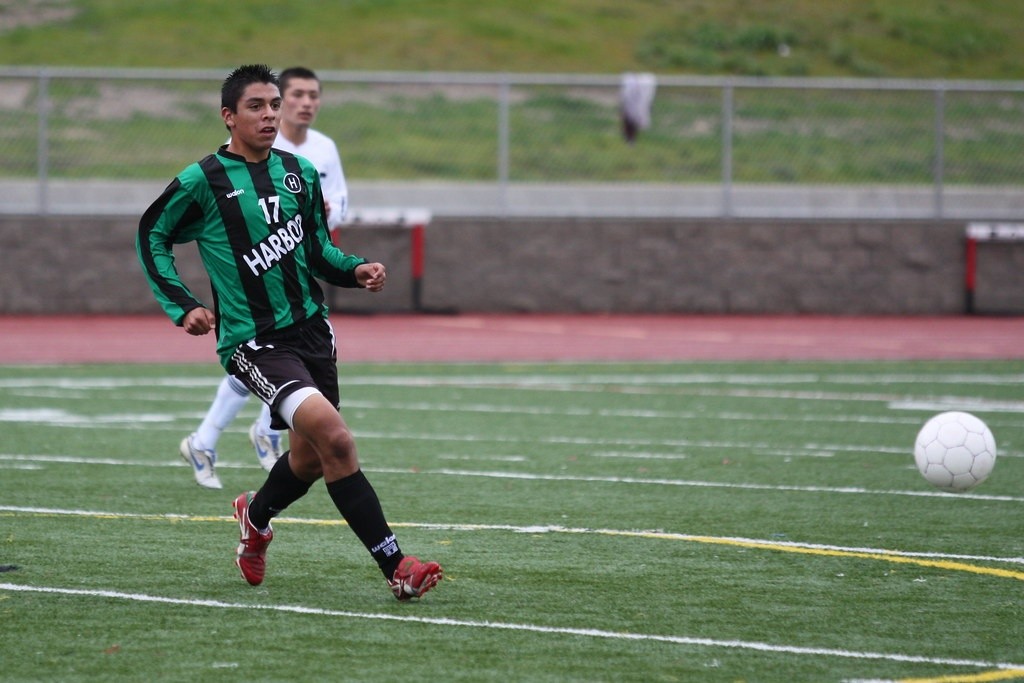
[134,65,442,602]
[180,66,349,489]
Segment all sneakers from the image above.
[250,421,284,473]
[384,556,443,601]
[232,491,274,587]
[179,433,222,489]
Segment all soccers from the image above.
[913,411,999,494]
